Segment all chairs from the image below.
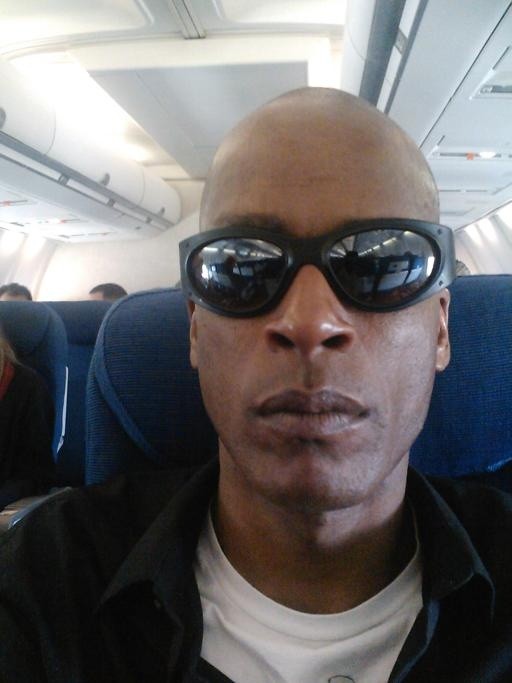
[0,271,512,534]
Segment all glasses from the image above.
[177,218,458,321]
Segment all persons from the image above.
[0,87,512,683]
[0,333,55,512]
[0,283,128,301]
